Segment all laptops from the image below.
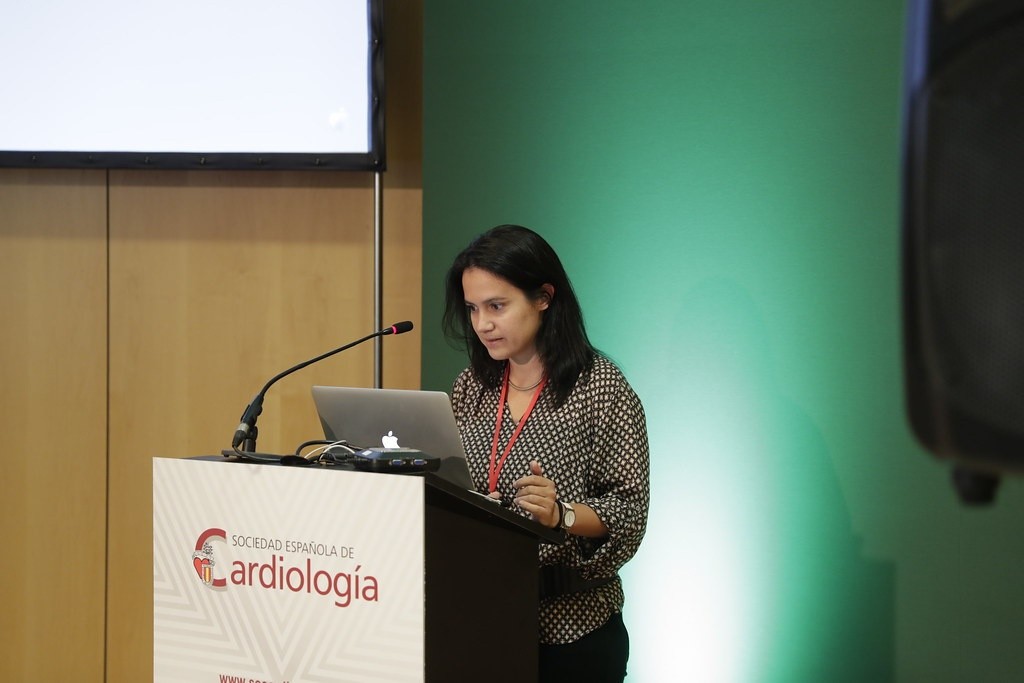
[309,386,476,492]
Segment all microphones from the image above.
[231,321,414,448]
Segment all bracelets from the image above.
[547,499,564,530]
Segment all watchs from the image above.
[554,502,576,532]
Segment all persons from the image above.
[441,225,651,683]
